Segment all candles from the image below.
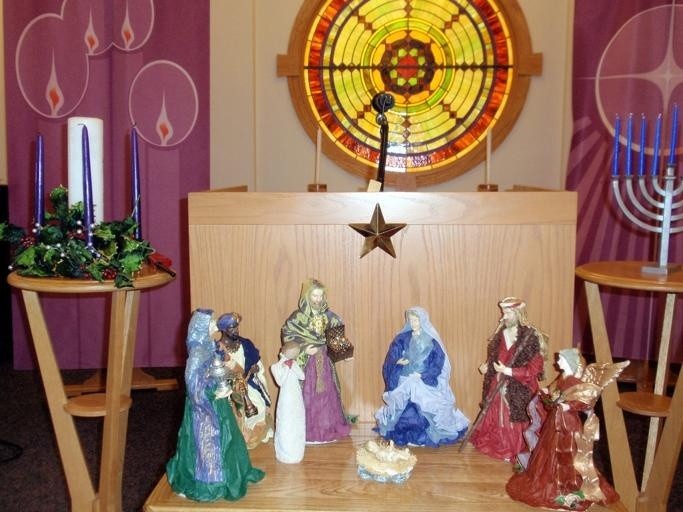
[485,123,492,184]
[611,98,680,179]
[315,124,322,184]
[25,121,141,247]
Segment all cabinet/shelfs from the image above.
[576,259,681,511]
[6,255,177,512]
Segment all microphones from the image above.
[372,93,395,113]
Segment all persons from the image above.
[215,309,274,450]
[162,308,266,504]
[268,337,314,468]
[466,295,547,461]
[502,347,631,508]
[277,274,357,446]
[369,306,469,444]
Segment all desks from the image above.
[141,420,627,512]
